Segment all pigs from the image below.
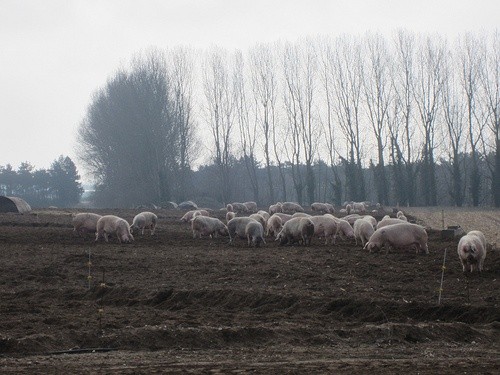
[71,201,406,248]
[363,223,429,256]
[457,230,486,273]
